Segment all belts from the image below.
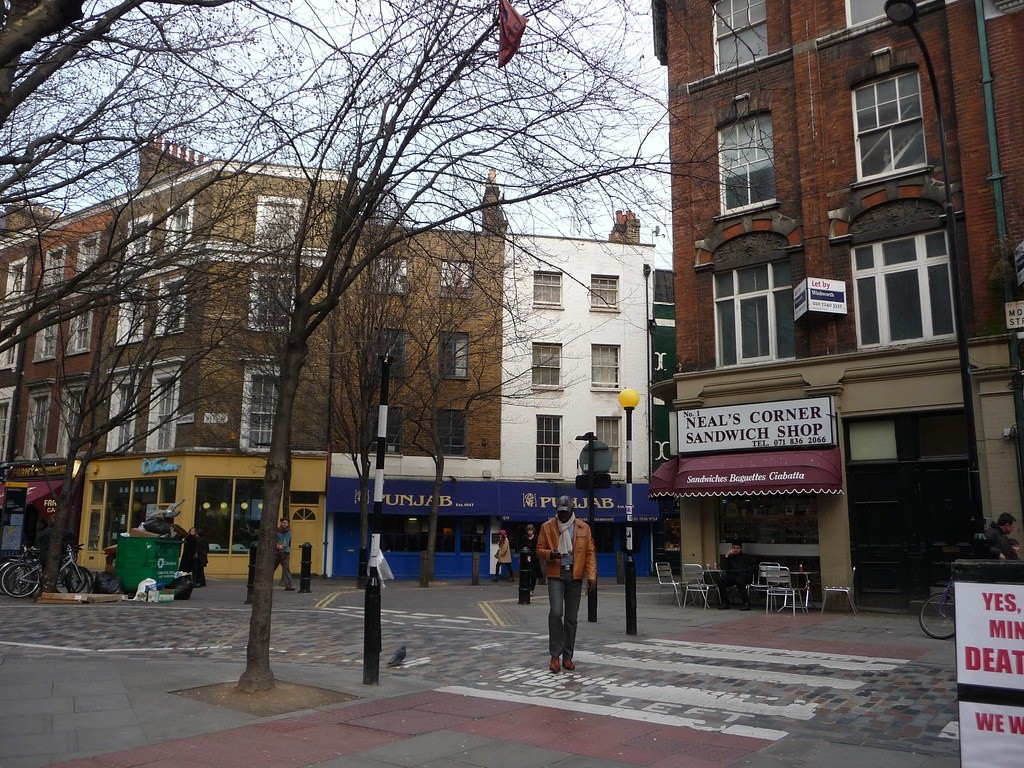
[560,565,573,570]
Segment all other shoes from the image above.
[719,602,731,609]
[492,576,498,582]
[550,657,561,673]
[285,585,296,592]
[562,658,575,670]
[740,603,751,610]
[507,577,514,582]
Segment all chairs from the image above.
[746,562,802,616]
[820,567,857,614]
[655,562,689,607]
[682,564,716,609]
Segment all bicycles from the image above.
[919,570,959,640]
[0,544,94,599]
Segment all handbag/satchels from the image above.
[494,549,500,558]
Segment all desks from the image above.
[700,568,725,607]
[787,570,819,617]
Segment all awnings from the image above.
[0,480,64,519]
[648,446,845,500]
[327,477,659,523]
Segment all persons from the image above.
[273,518,296,591]
[536,496,596,674]
[718,540,758,611]
[491,529,515,582]
[179,527,209,588]
[525,524,537,597]
[978,512,1020,560]
[39,520,53,571]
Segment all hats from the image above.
[557,495,573,513]
[499,530,507,535]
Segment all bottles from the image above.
[714,563,717,570]
[799,564,803,572]
[706,564,710,570]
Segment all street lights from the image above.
[619,388,639,634]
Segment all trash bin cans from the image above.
[113,532,186,593]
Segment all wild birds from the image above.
[386,644,406,666]
